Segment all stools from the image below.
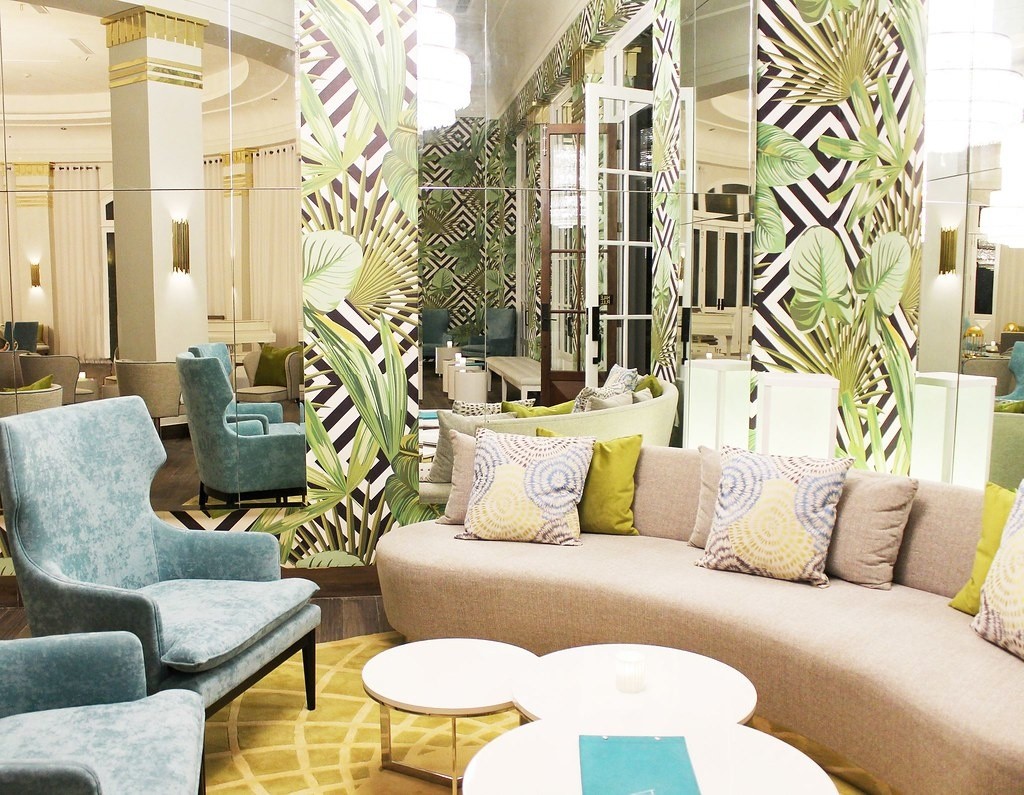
[75,377,98,402]
[435,346,487,404]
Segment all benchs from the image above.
[487,356,541,401]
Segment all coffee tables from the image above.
[362,637,842,795]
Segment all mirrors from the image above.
[417,0,756,507]
[0,0,302,510]
[949,141,1023,489]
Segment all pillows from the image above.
[688,445,918,590]
[435,428,644,537]
[419,365,663,483]
[253,343,302,387]
[945,478,1024,662]
[3,373,54,391]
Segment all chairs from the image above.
[0,395,322,795]
[37,323,50,350]
[0,383,64,416]
[421,308,448,357]
[188,344,287,435]
[18,353,81,405]
[1001,331,1024,355]
[113,347,186,433]
[13,321,38,351]
[962,357,1011,396]
[461,307,515,357]
[178,343,306,506]
[234,349,304,424]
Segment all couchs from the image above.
[418,376,679,506]
[374,444,1023,795]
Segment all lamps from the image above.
[30,256,40,287]
[962,326,985,358]
